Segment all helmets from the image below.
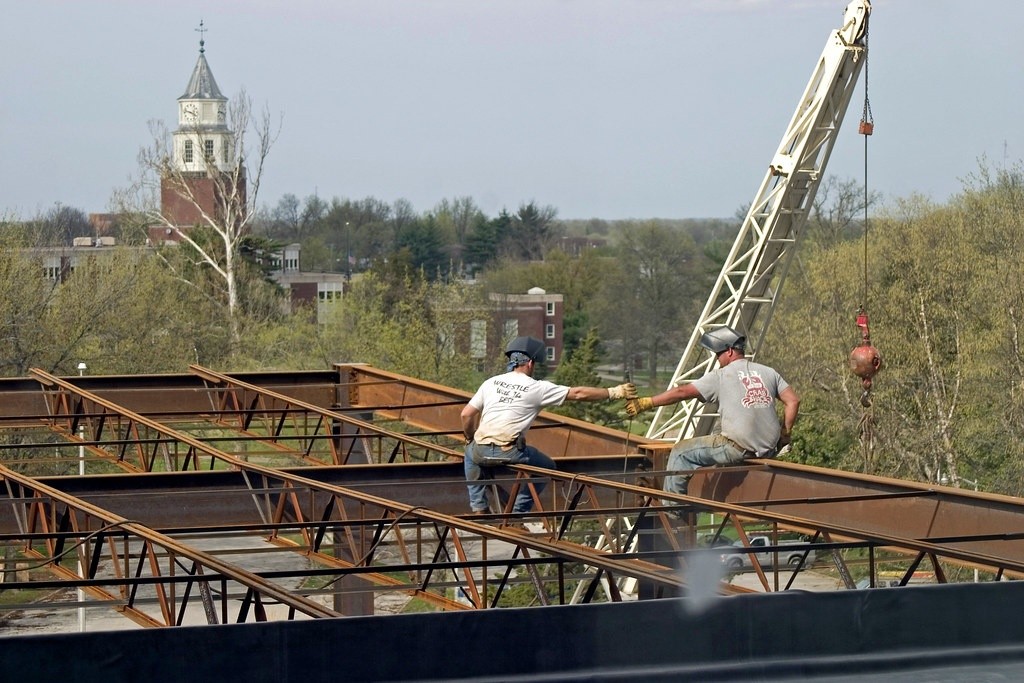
[504,336,546,362]
[698,325,746,352]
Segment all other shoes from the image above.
[645,496,681,520]
[511,521,529,531]
[477,510,492,514]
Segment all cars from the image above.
[697,534,735,547]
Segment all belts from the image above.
[729,440,755,456]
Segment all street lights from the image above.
[78,361,88,636]
[345,222,350,284]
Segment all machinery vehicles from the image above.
[850,571,937,591]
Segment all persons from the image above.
[625,326,799,520]
[460,336,639,532]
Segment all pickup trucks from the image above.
[713,535,817,575]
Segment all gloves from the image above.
[776,427,791,451]
[624,396,655,417]
[606,382,638,401]
